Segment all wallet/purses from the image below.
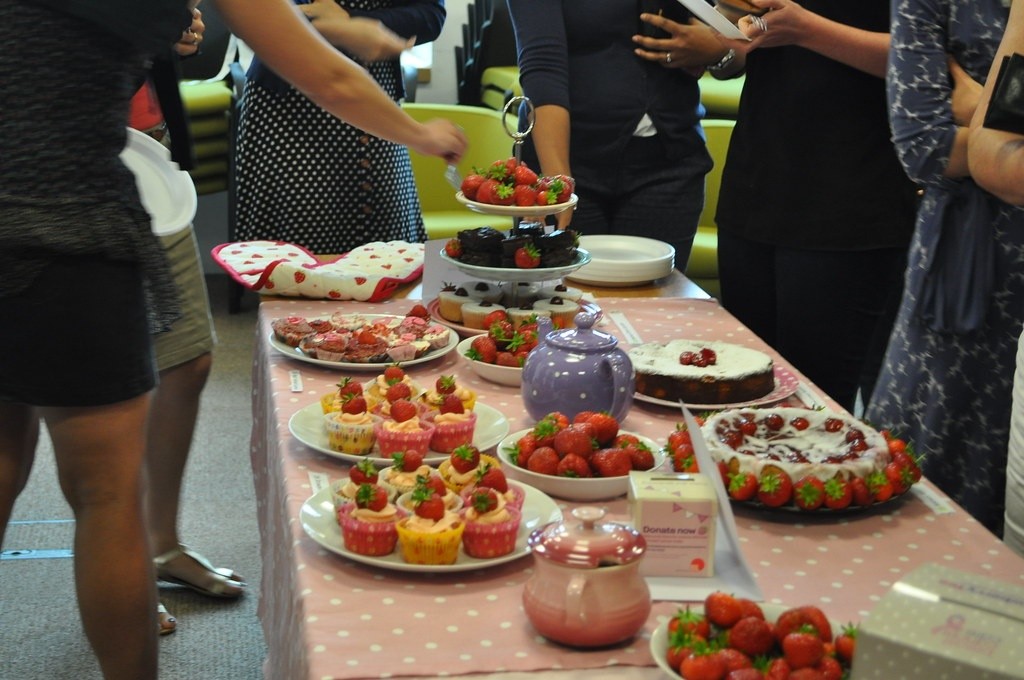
[983,51,1024,135]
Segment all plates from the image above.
[440,247,592,281]
[426,298,604,337]
[455,191,578,215]
[678,0,751,41]
[120,127,197,236]
[567,234,676,287]
[728,483,912,517]
[633,359,801,411]
[269,314,460,371]
[299,476,564,573]
[288,399,511,467]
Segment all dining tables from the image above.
[248,253,1024,680]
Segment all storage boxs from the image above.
[628,470,718,579]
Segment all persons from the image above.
[118,8,248,635]
[967,0,1024,559]
[0,0,470,680]
[506,0,716,276]
[860,0,1024,542]
[228,0,446,315]
[708,0,924,418]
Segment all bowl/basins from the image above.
[497,428,665,501]
[456,333,523,387]
[650,603,848,680]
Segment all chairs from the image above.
[122,0,747,304]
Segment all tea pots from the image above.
[521,312,635,423]
[523,506,652,646]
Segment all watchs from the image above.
[706,48,736,70]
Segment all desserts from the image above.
[321,366,477,458]
[271,304,449,361]
[456,221,580,268]
[440,280,584,330]
[333,444,525,564]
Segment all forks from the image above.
[752,16,767,31]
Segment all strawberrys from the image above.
[517,411,655,477]
[461,158,575,205]
[473,310,565,367]
[516,247,540,268]
[668,593,853,680]
[446,239,465,258]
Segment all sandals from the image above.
[154,542,246,599]
[156,601,178,636]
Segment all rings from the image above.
[194,33,198,46]
[667,52,671,63]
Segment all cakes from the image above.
[629,338,776,406]
[669,403,921,509]
